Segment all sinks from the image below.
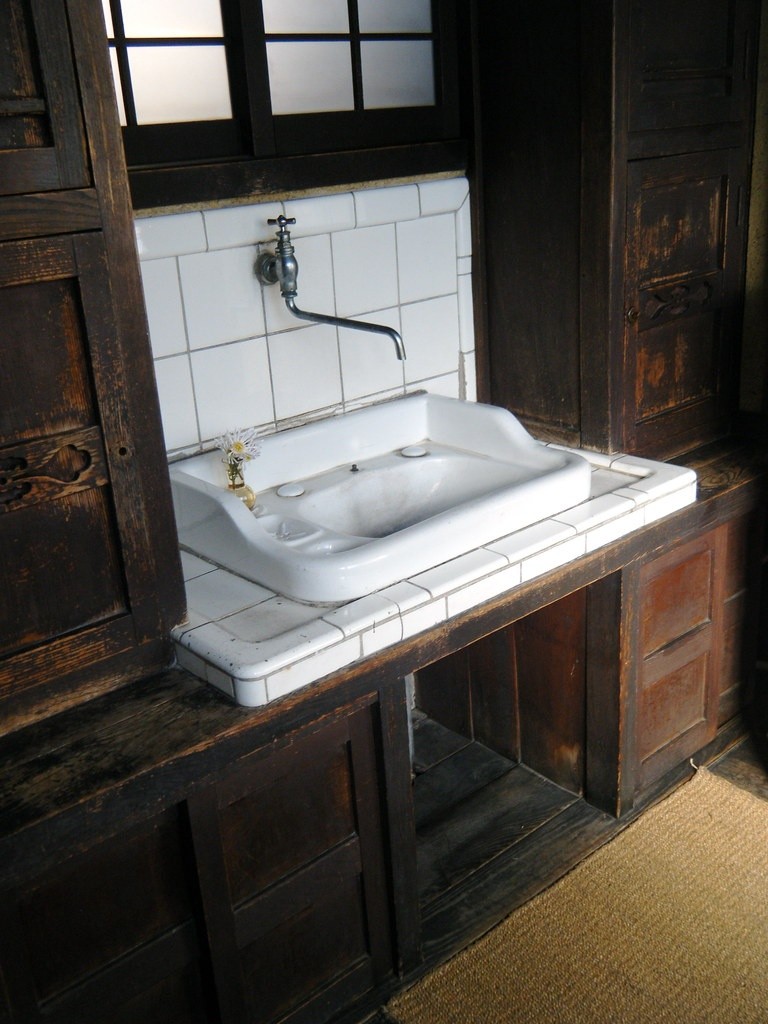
[236,450,592,604]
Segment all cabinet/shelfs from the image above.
[1,0,767,1024]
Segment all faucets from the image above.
[255,215,407,360]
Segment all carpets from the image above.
[381,767,768,1024]
